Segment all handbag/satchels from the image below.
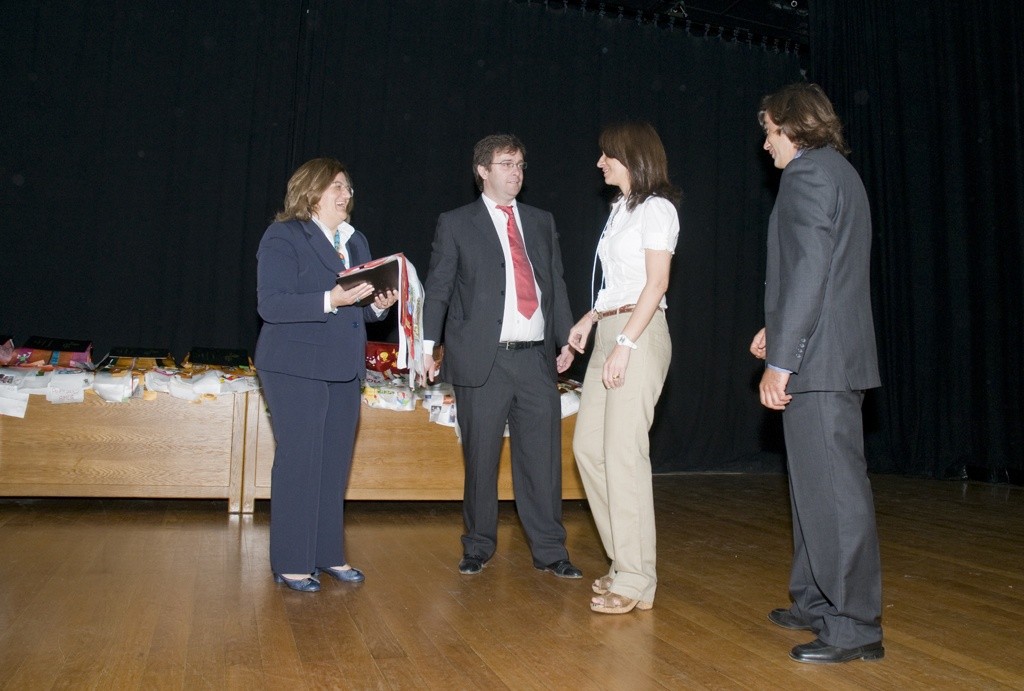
[428,345,444,384]
[366,341,408,374]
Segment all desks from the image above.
[0,359,590,514]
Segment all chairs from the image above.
[22,337,250,366]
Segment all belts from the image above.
[498,340,544,350]
[598,305,662,320]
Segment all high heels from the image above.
[592,575,614,594]
[590,591,653,613]
[273,572,320,592]
[317,566,365,582]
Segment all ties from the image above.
[496,205,539,320]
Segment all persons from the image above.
[415,134,584,579]
[568,119,680,614]
[749,80,885,663]
[254,158,399,591]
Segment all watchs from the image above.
[614,334,638,351]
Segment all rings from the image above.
[355,297,360,303]
[612,375,619,379]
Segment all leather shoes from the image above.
[457,555,485,574]
[788,639,884,664]
[533,561,583,578]
[767,609,822,634]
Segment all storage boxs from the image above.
[365,341,411,374]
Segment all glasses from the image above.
[331,182,353,197]
[490,160,528,170]
